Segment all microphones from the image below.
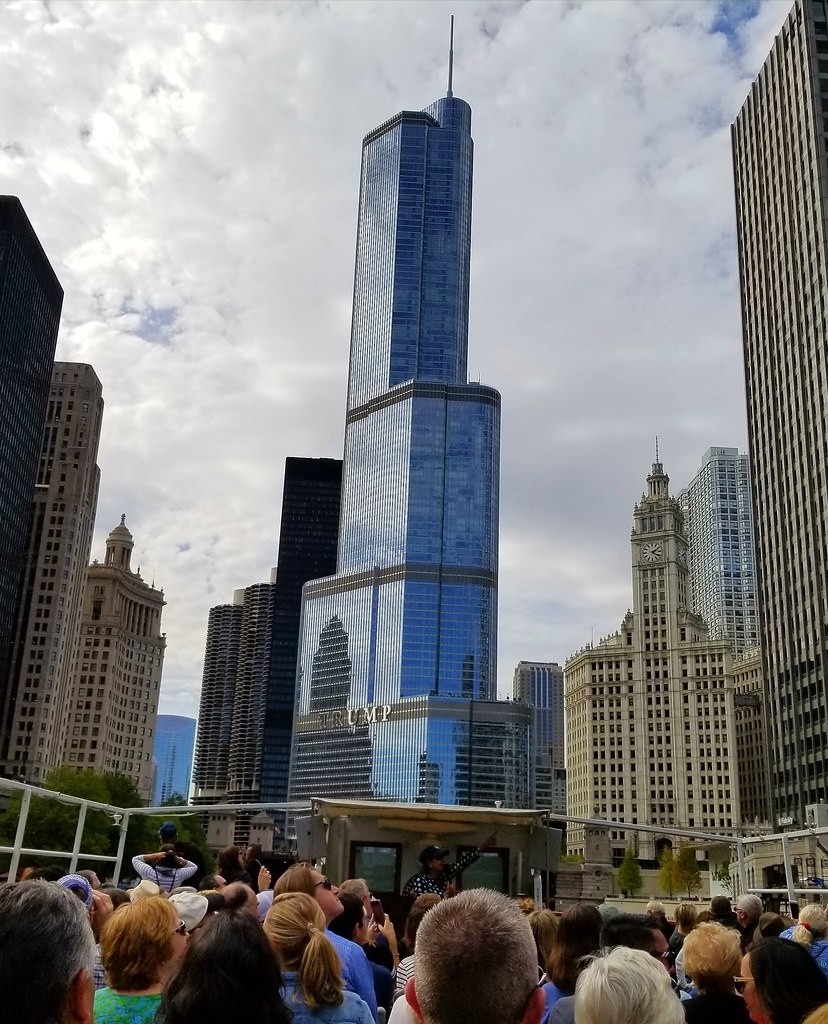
[443,864,453,885]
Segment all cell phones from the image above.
[371,899,384,932]
[256,860,262,869]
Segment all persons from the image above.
[0,822,828,1024]
[215,846,251,882]
[574,947,686,1024]
[220,882,260,919]
[156,908,293,1023]
[405,887,547,1024]
[1,878,98,1024]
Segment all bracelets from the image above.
[478,846,481,851]
[393,953,400,956]
[180,857,184,864]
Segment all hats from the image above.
[158,823,178,841]
[753,912,795,944]
[596,903,621,933]
[419,845,450,865]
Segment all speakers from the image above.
[293,815,327,863]
[527,826,562,872]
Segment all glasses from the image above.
[734,975,755,996]
[518,965,548,1021]
[168,919,186,938]
[314,878,332,891]
[433,856,445,860]
[733,907,741,911]
[649,951,675,969]
